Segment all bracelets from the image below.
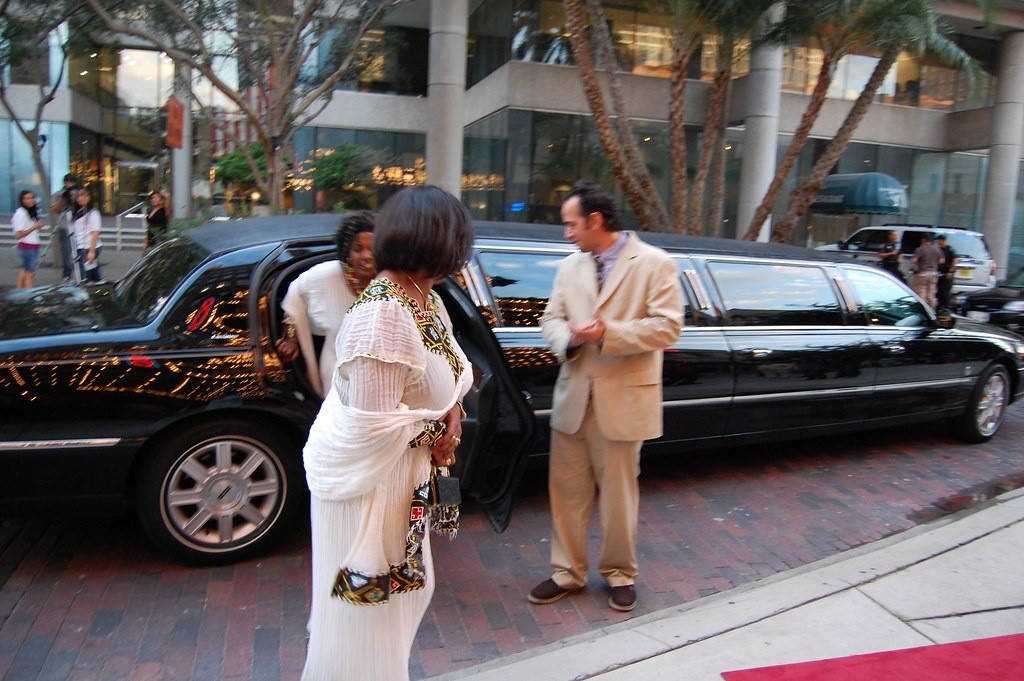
[24,226,36,235]
[949,271,954,273]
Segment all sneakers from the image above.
[608,585,637,611]
[527,578,571,604]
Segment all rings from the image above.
[453,436,456,439]
[446,459,451,466]
[282,342,286,345]
[456,438,460,444]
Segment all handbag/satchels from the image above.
[429,474,462,507]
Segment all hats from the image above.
[934,234,946,240]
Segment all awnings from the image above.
[810,172,910,228]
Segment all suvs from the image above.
[813,223,998,307]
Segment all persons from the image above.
[528,184,683,611]
[72,186,103,281]
[12,191,45,289]
[300,185,473,681]
[880,231,907,284]
[934,235,957,311]
[49,174,79,282]
[912,234,944,308]
[275,211,376,400]
[145,192,166,248]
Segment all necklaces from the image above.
[402,270,426,301]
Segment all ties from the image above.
[595,261,606,294]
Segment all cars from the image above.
[961,267,1024,341]
[0,216,1024,568]
